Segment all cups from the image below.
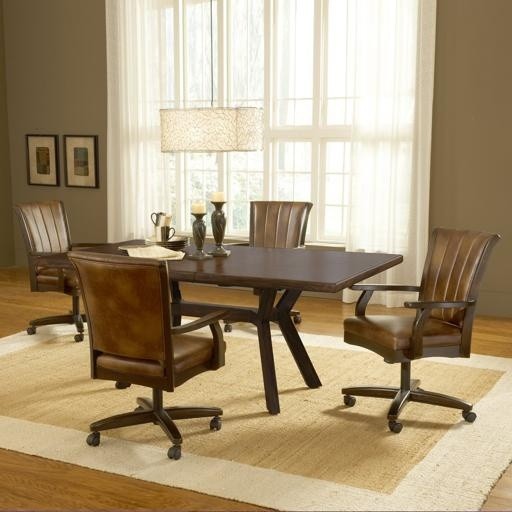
[151,212,175,242]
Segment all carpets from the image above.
[2,320,510,511]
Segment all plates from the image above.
[118,236,188,256]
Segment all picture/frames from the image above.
[26,134,99,189]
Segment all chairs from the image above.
[216,202,315,332]
[17,200,142,344]
[68,252,229,460]
[342,226,500,434]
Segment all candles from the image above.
[212,192,224,201]
[190,202,207,214]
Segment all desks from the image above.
[75,237,404,415]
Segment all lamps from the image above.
[159,2,263,152]
